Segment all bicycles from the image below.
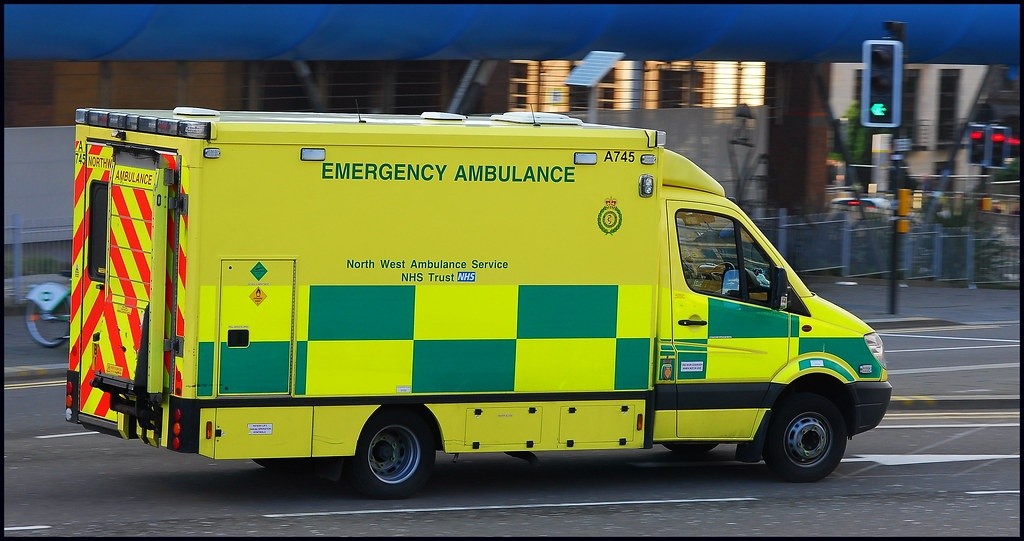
[24,281,71,349]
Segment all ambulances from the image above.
[62,107,892,499]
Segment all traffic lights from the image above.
[986,126,1011,169]
[860,40,903,127]
[965,124,989,166]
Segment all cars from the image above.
[831,197,891,210]
